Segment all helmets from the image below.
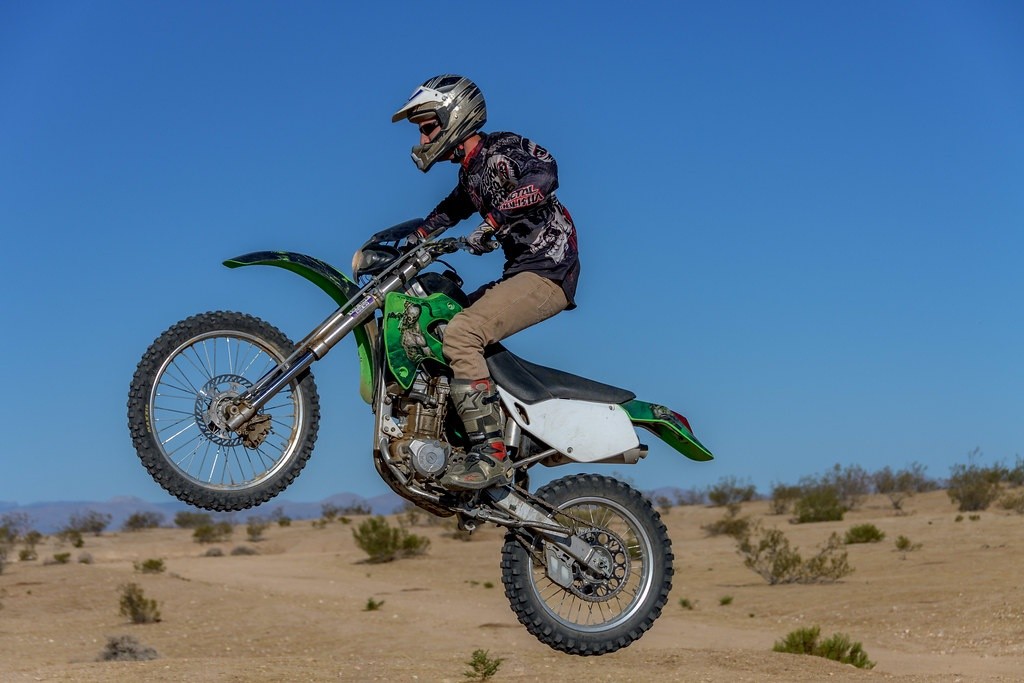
[392,74,487,173]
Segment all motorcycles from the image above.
[127,217,714,657]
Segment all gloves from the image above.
[466,219,496,253]
[404,231,424,247]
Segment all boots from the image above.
[442,376,514,489]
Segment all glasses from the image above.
[418,120,439,136]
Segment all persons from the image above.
[392,73,581,489]
